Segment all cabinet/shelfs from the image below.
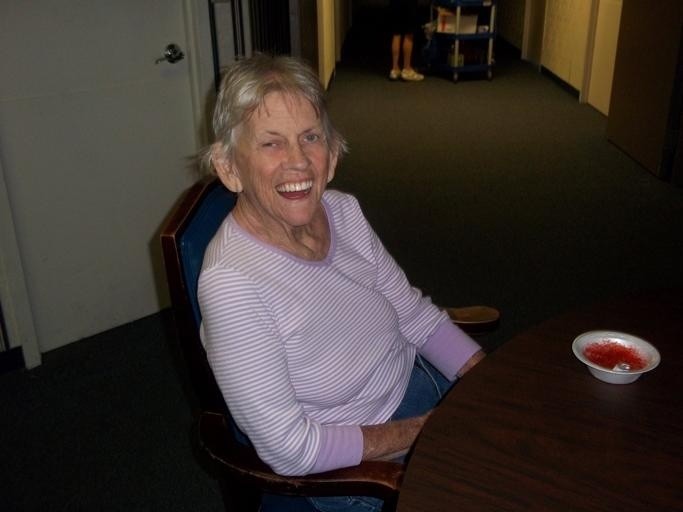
[424,0,498,84]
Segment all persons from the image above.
[389,32,424,81]
[197,51,487,512]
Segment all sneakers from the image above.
[389,69,425,82]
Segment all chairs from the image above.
[162,169,503,510]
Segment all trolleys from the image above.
[410,1,498,85]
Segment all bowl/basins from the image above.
[570,329,662,386]
